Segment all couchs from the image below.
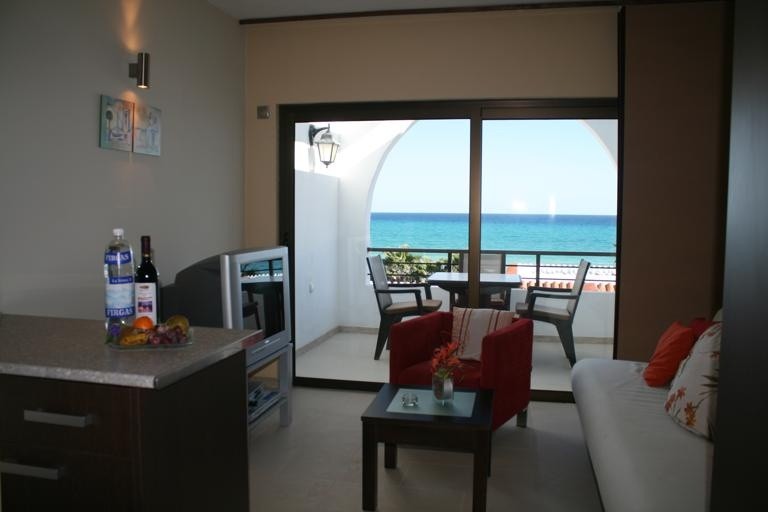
[571,303,730,511]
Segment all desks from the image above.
[359,380,496,511]
[425,270,524,312]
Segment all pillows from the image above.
[643,321,699,388]
[664,318,722,440]
[450,306,514,364]
[690,313,718,333]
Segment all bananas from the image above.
[120,333,147,345]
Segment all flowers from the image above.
[428,334,474,386]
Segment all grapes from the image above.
[146,324,187,345]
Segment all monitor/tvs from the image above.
[160,245,292,367]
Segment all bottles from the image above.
[103,226,134,332]
[134,235,159,329]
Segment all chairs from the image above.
[454,250,510,307]
[385,307,534,442]
[511,258,592,367]
[366,253,444,363]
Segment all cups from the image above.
[398,391,419,408]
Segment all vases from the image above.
[429,369,457,409]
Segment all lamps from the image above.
[306,123,340,167]
[125,48,153,92]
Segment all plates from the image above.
[106,321,194,349]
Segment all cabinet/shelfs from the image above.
[1,309,264,511]
[245,340,297,456]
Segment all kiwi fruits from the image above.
[164,315,190,334]
